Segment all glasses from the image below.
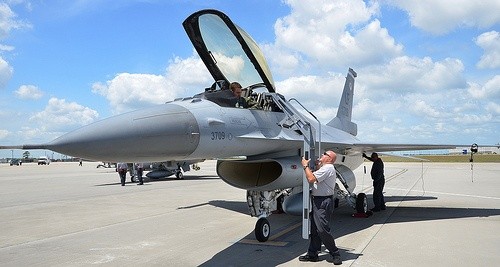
[324,151,330,158]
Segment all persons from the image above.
[78,160,83,166]
[362,153,385,211]
[298,150,342,264]
[230,82,248,108]
[135,162,143,185]
[117,162,128,186]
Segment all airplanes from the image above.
[0,9,500,242]
[115,159,206,182]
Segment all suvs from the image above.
[38,156,51,165]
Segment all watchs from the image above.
[303,165,308,170]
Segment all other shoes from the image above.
[299,255,316,262]
[369,206,381,212]
[380,205,386,210]
[332,254,343,266]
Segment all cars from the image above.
[10,160,22,165]
